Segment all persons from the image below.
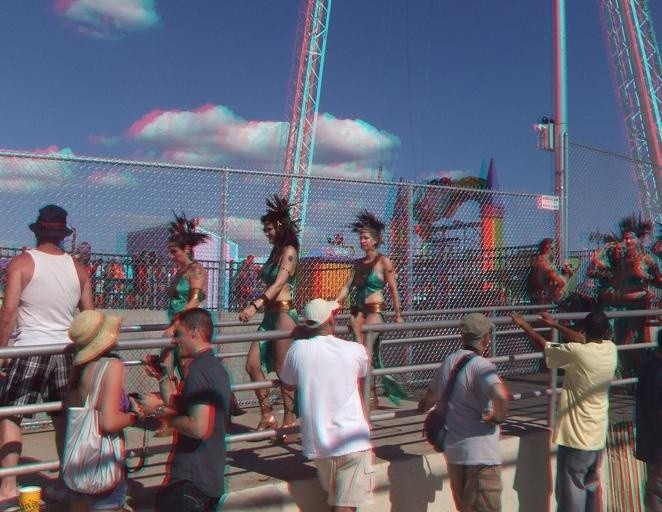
[418,313,510,512]
[280,299,376,512]
[635,329,662,512]
[334,209,404,365]
[230,255,262,310]
[150,212,211,438]
[137,308,232,512]
[60,311,142,512]
[238,198,299,433]
[507,310,617,512]
[76,241,169,309]
[0,205,95,511]
[526,209,661,344]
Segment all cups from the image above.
[18,484,42,512]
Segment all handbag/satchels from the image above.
[421,407,446,452]
[59,405,128,495]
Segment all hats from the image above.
[27,203,75,244]
[459,313,495,341]
[67,310,123,368]
[303,298,340,330]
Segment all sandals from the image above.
[154,423,173,436]
[368,386,380,411]
[282,392,297,431]
[253,392,279,432]
